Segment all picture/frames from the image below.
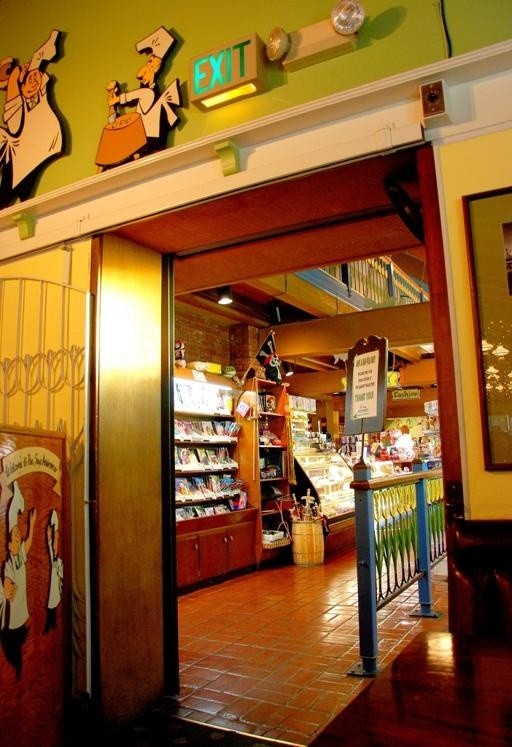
[461,184,512,470]
[1,425,74,746]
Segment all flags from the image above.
[255,333,282,381]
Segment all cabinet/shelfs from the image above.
[289,397,320,451]
[174,365,291,591]
[296,451,362,555]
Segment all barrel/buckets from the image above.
[292,520,325,566]
